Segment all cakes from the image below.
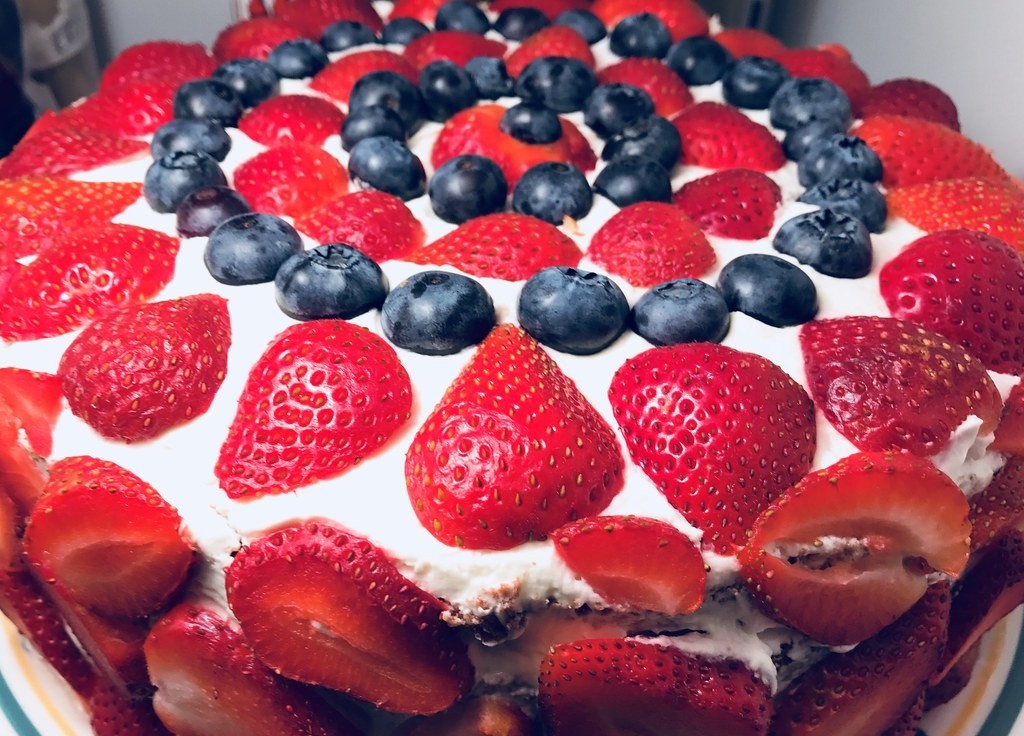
[1,0,1024,735]
